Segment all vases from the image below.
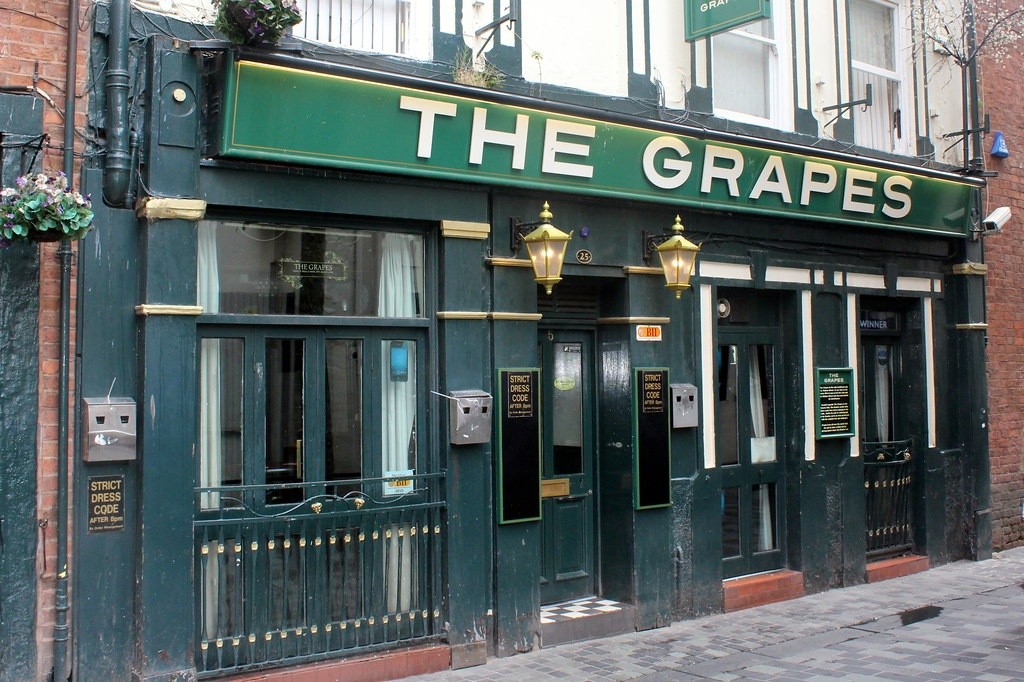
[27,226,64,243]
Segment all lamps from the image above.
[510,201,574,296]
[642,214,702,300]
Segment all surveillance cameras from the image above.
[983,206,1012,232]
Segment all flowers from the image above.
[0,171,95,248]
[209,0,303,48]
